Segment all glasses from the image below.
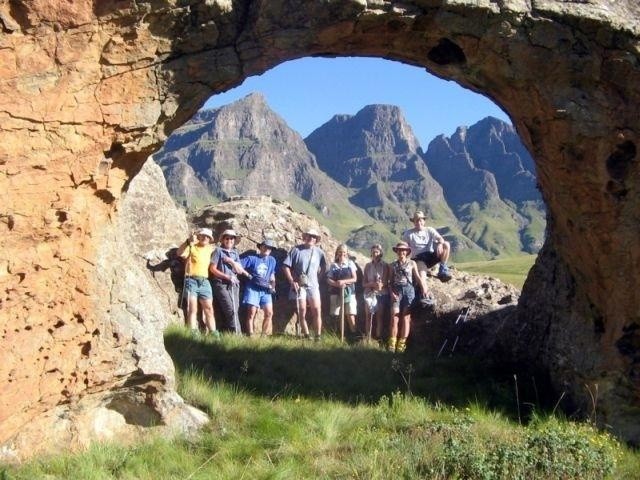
[184,276,209,280]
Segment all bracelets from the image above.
[186,239,191,248]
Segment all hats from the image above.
[392,242,411,256]
[219,229,241,246]
[196,228,214,242]
[257,240,276,250]
[336,286,351,307]
[302,227,321,245]
[411,211,427,222]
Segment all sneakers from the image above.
[438,264,452,278]
[304,333,321,341]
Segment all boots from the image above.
[389,337,407,354]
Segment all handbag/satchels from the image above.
[251,276,271,289]
[401,282,425,316]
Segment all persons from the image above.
[208,229,243,337]
[177,228,223,342]
[326,244,358,345]
[361,244,389,342]
[240,239,277,339]
[388,241,424,355]
[282,229,326,346]
[402,211,452,310]
